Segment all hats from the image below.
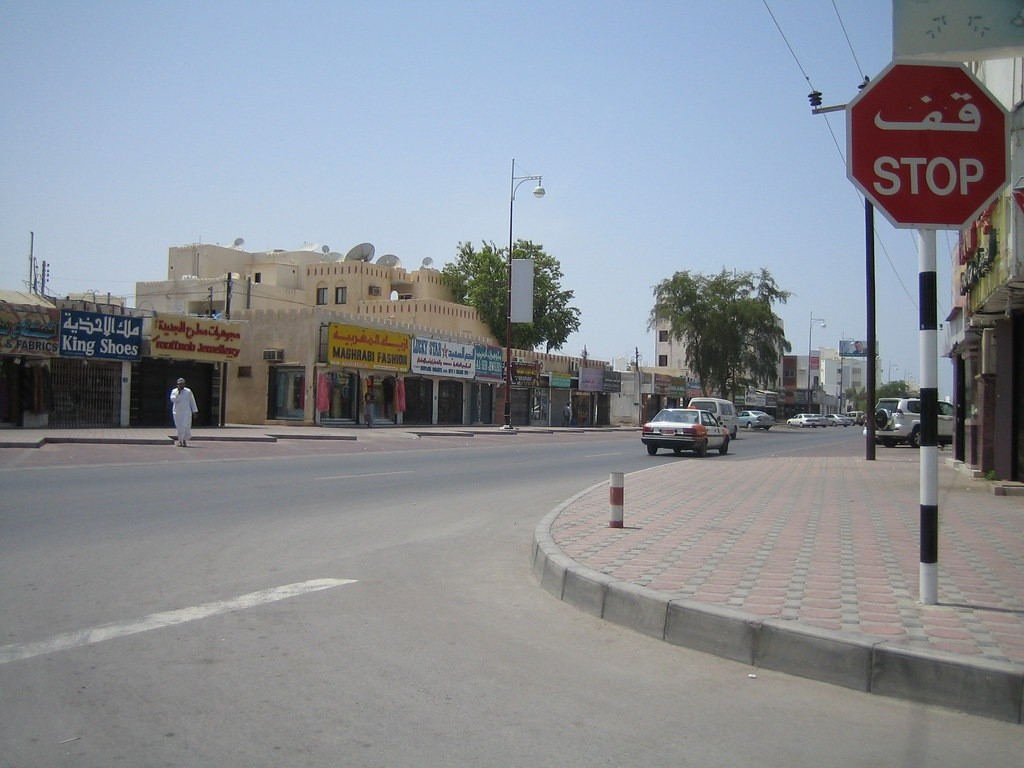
[177,378,185,384]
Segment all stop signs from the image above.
[846,60,1011,231]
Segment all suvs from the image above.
[862,398,953,448]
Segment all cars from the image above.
[786,414,819,428]
[825,413,852,427]
[738,410,774,432]
[641,406,730,458]
[812,414,827,428]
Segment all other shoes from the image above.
[178,442,182,447]
[183,443,186,447]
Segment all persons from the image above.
[853,341,867,353]
[170,378,199,447]
[561,402,572,427]
[363,386,376,427]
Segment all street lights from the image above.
[888,361,916,386]
[839,333,856,412]
[806,311,827,412]
[503,158,545,428]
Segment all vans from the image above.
[846,411,864,426]
[687,397,737,439]
